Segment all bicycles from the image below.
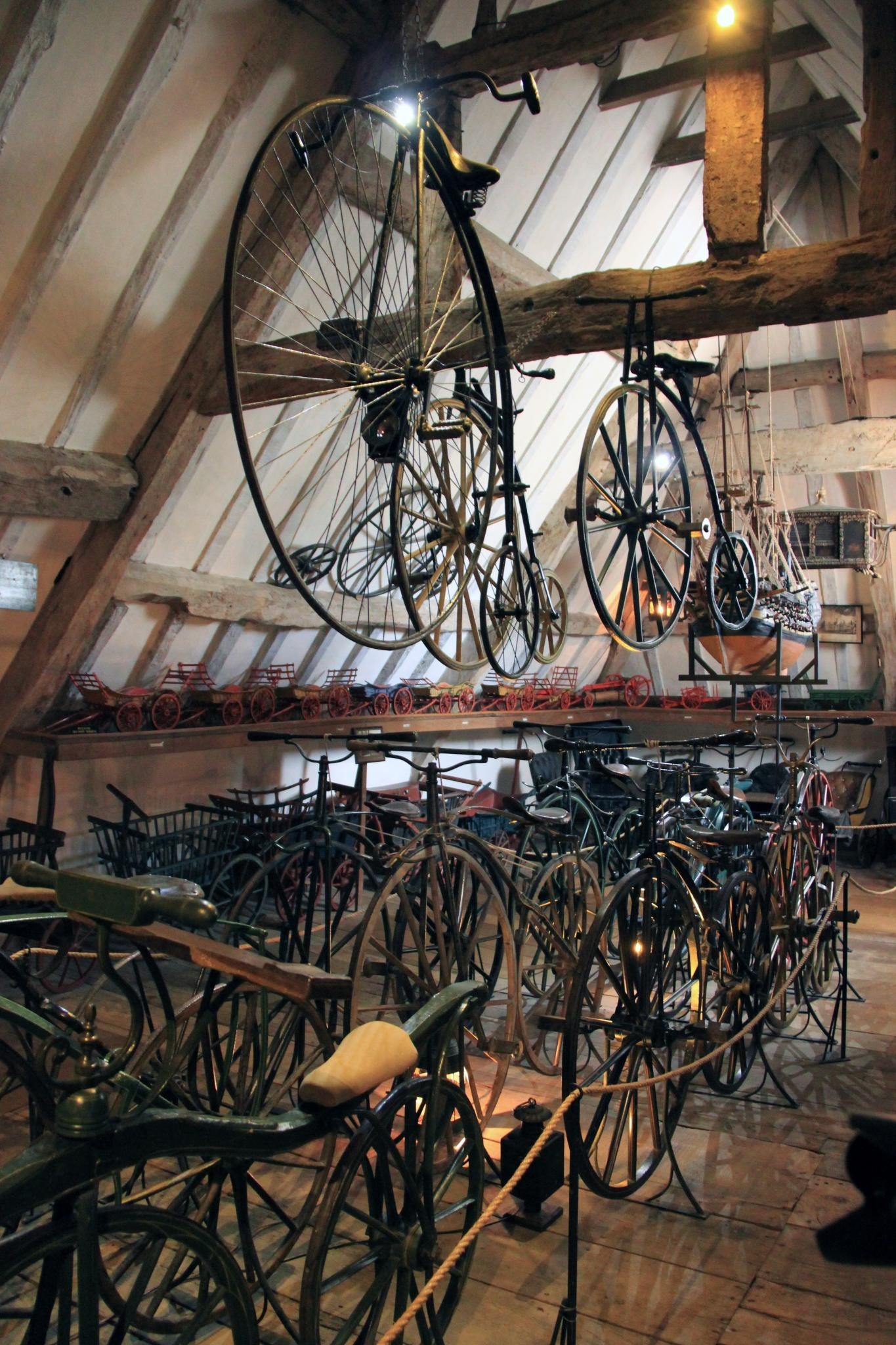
[2,40,885,1344]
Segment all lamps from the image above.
[646,585,686,624]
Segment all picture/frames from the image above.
[812,603,863,644]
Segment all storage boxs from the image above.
[775,501,880,571]
